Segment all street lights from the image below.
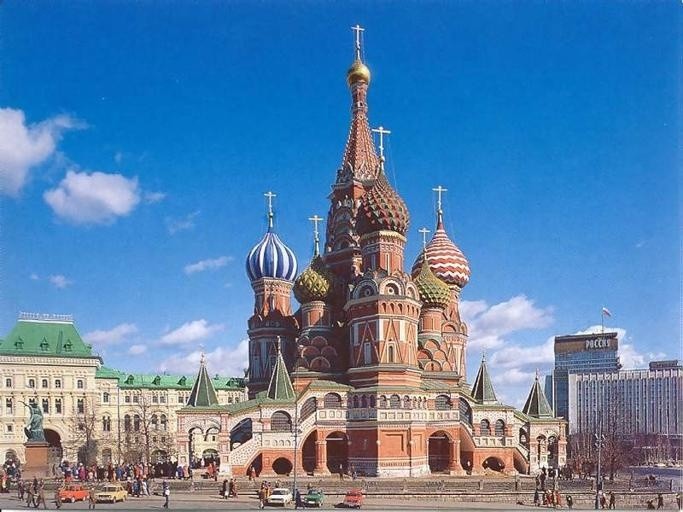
[593,421,604,508]
[288,404,302,506]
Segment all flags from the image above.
[602,306,616,320]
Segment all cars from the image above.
[303,489,325,507]
[266,487,293,506]
[342,487,363,509]
[59,484,88,503]
[93,482,129,503]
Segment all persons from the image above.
[655,495,664,510]
[0,458,22,493]
[248,463,258,482]
[294,488,305,509]
[221,475,238,500]
[89,486,95,509]
[162,478,171,509]
[339,463,344,481]
[208,461,217,481]
[55,491,62,509]
[53,451,190,496]
[257,480,279,510]
[17,476,47,509]
[599,490,616,510]
[533,464,573,510]
